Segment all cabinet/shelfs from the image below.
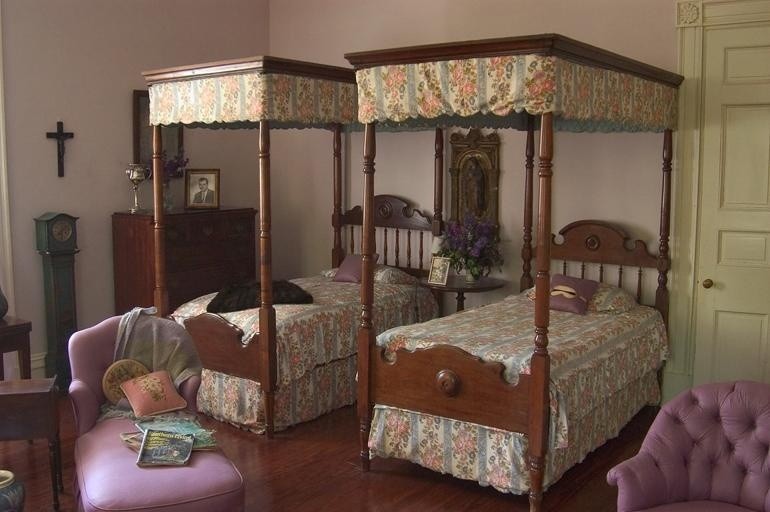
[112,214,256,317]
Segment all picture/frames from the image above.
[131,89,220,210]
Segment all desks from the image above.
[0,314,65,511]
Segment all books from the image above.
[119,408,217,469]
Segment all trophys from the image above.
[124,162,154,215]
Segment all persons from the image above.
[193,178,214,204]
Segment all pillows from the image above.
[101,357,188,419]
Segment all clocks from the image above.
[34,211,80,253]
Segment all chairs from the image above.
[66,312,245,512]
[605,380,770,512]
